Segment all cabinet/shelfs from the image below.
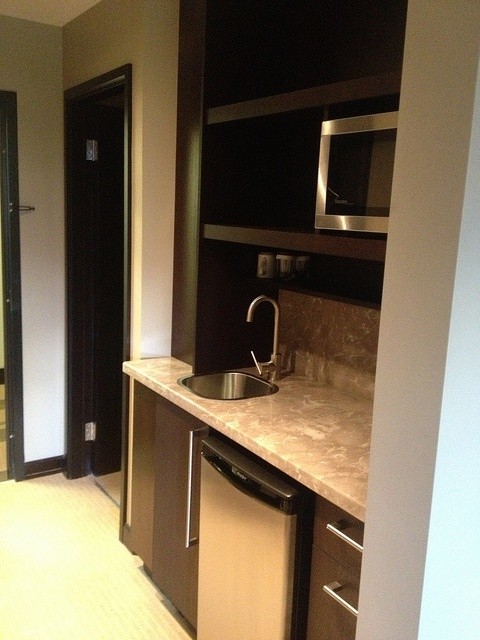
[303,493,365,640]
[146,392,212,630]
[251,256,383,311]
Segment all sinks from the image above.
[183,372,274,401]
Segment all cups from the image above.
[305,348,329,386]
[276,253,296,282]
[255,251,277,280]
[293,255,309,280]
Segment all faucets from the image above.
[246,295,283,382]
